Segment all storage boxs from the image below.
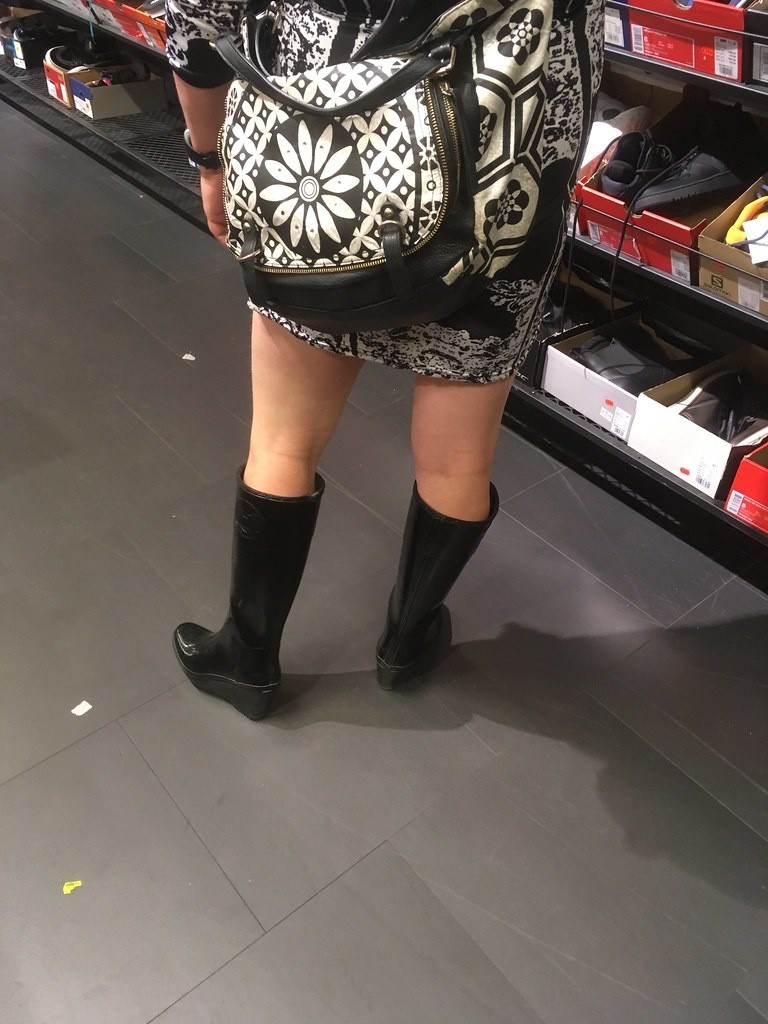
[0,0,168,119]
[516,0,768,535]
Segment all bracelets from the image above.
[183,128,221,170]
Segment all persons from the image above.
[163,0,605,722]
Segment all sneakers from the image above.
[601,99,754,213]
[83,62,152,88]
[46,36,122,72]
[669,366,767,450]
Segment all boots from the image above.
[172,464,324,720]
[374,478,500,691]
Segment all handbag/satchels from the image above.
[219,40,482,333]
